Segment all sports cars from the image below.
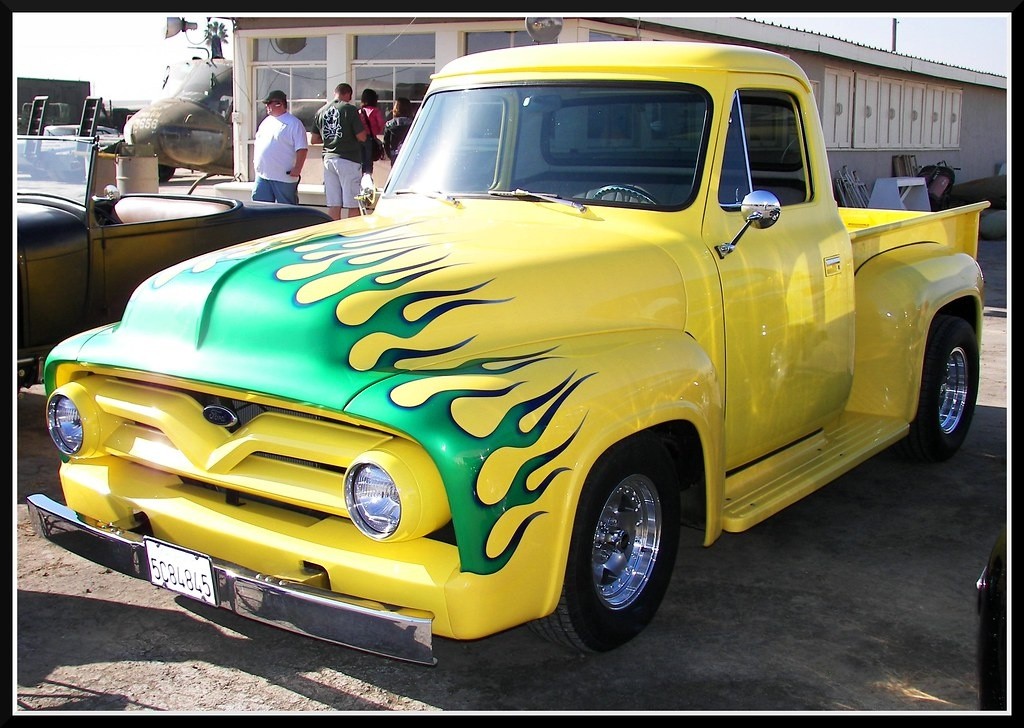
[16,135,333,392]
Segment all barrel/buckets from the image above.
[115,155,160,197]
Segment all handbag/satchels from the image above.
[372,138,385,161]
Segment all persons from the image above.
[252,90,309,205]
[357,89,385,177]
[311,83,367,220]
[383,97,413,167]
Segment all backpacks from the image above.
[387,117,413,154]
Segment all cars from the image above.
[38,126,126,180]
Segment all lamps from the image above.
[525,17,563,43]
[161,17,210,46]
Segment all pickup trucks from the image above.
[27,40,992,665]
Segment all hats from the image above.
[262,89,286,103]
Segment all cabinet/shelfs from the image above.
[867,176,932,213]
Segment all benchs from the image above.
[110,197,229,224]
[517,171,807,208]
